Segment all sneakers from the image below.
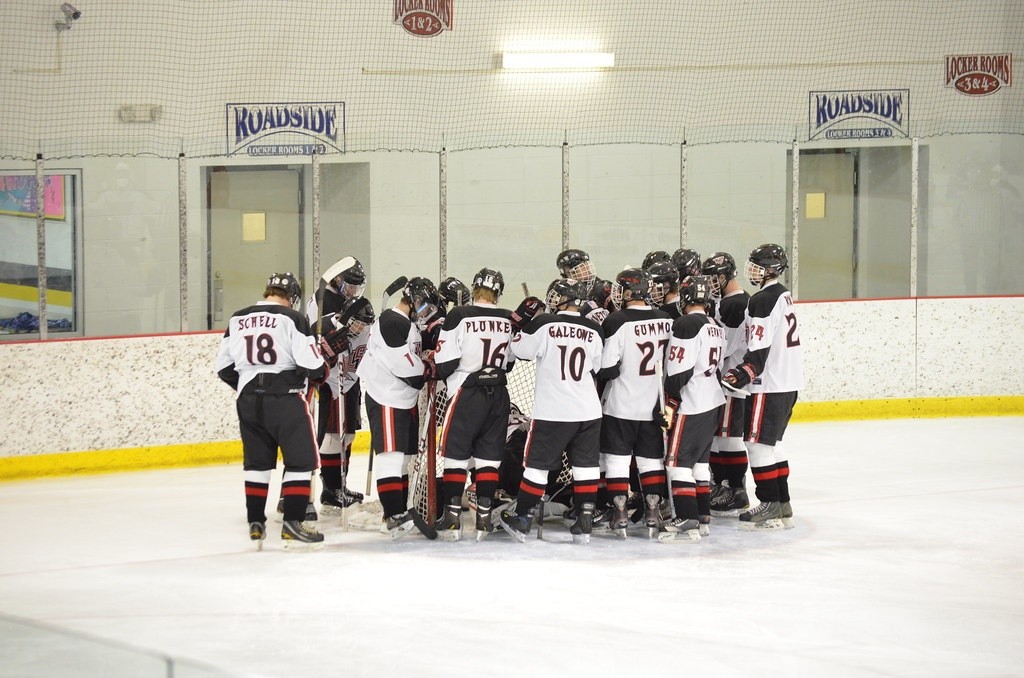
[380,485,796,542]
[249,487,363,550]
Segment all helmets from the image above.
[556,249,596,292]
[472,268,504,297]
[267,273,301,312]
[339,296,375,340]
[439,276,470,306]
[402,277,438,307]
[744,243,788,286]
[610,268,652,310]
[589,280,615,312]
[641,247,736,316]
[336,258,367,301]
[546,279,586,309]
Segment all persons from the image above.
[499,278,605,545]
[640,249,701,285]
[300,256,376,516]
[701,251,751,517]
[658,275,728,544]
[217,272,330,552]
[721,243,804,532]
[557,249,614,312]
[433,266,517,543]
[438,277,471,306]
[601,267,674,539]
[355,276,439,541]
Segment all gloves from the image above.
[722,361,757,392]
[310,360,332,384]
[652,392,679,429]
[422,352,438,379]
[511,297,545,327]
[320,327,351,361]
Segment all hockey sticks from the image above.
[336,296,369,532]
[306,256,356,403]
[366,275,409,496]
[407,327,441,540]
